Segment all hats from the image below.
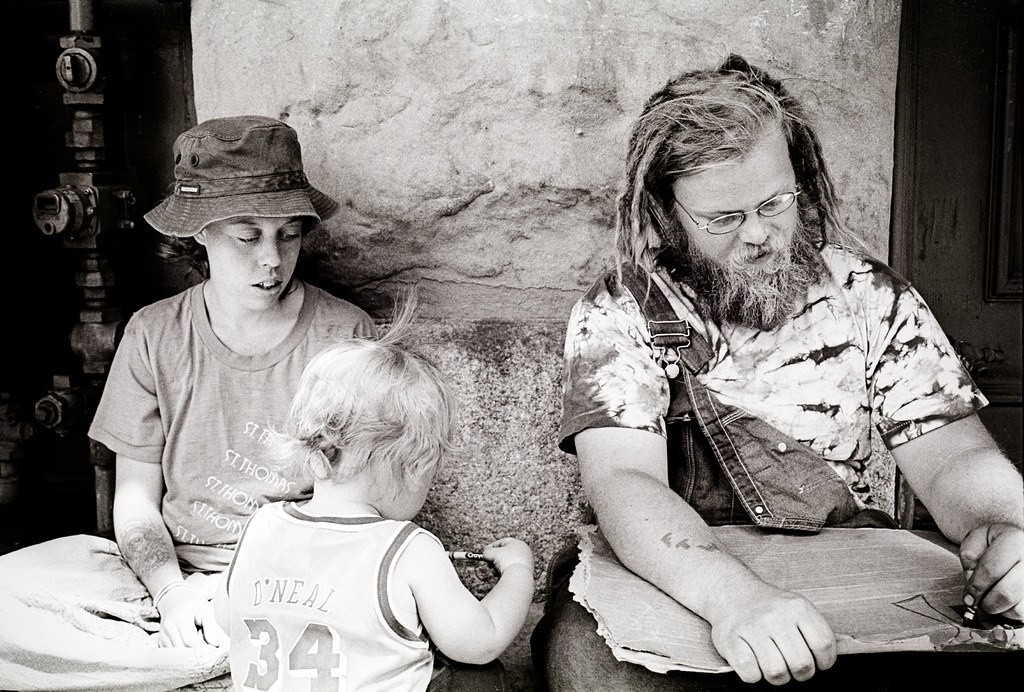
[141,115,340,237]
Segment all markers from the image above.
[447,551,485,560]
[962,605,978,628]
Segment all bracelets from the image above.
[152,579,182,608]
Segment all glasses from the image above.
[674,187,805,236]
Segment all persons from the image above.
[212,286,534,692]
[531,51,1024,692]
[0,115,378,692]
